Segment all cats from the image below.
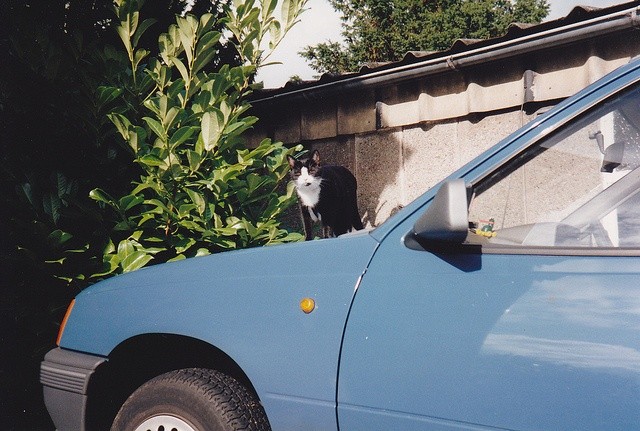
[286,149,365,242]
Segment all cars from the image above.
[37,49,639,431]
